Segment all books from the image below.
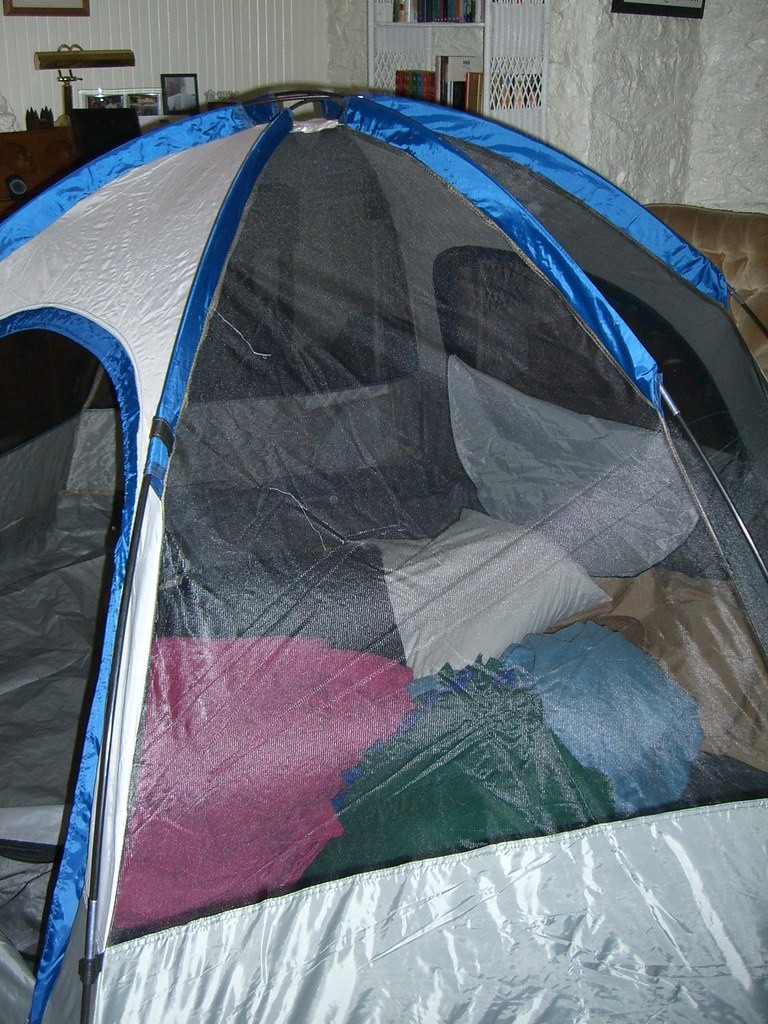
[394,56,484,118]
[373,0,485,24]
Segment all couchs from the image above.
[640,203,768,379]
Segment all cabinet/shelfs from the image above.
[0,128,75,223]
[367,0,547,142]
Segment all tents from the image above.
[0,80,768,1024]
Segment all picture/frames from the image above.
[161,73,201,115]
[3,0,91,17]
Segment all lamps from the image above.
[35,42,136,125]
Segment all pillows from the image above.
[383,507,614,678]
